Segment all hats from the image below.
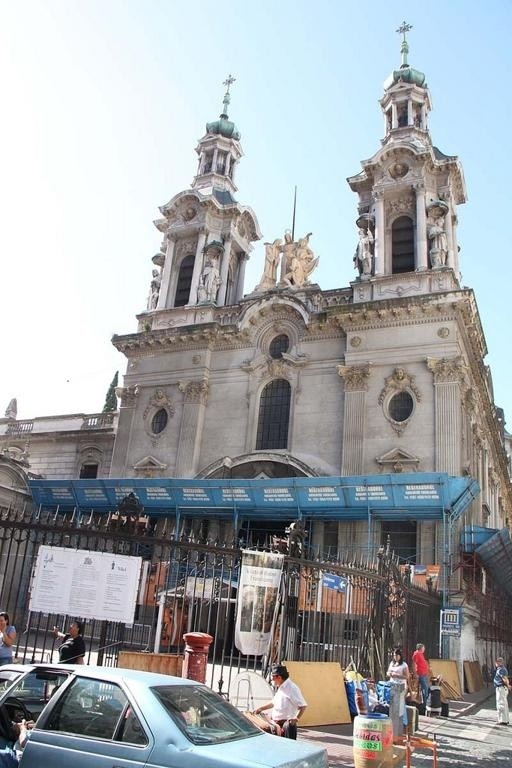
[272,666,288,676]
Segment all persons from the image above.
[427,215,449,267]
[53,619,85,664]
[261,232,314,290]
[146,268,161,310]
[389,366,412,389]
[493,657,511,725]
[151,388,170,407]
[1,612,17,666]
[354,227,375,273]
[253,665,309,740]
[200,257,223,304]
[365,643,431,737]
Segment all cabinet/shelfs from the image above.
[114,650,184,705]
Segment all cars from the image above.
[0,662,328,767]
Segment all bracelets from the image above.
[295,717,300,722]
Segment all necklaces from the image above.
[11,684,61,764]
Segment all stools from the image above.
[424,705,443,717]
